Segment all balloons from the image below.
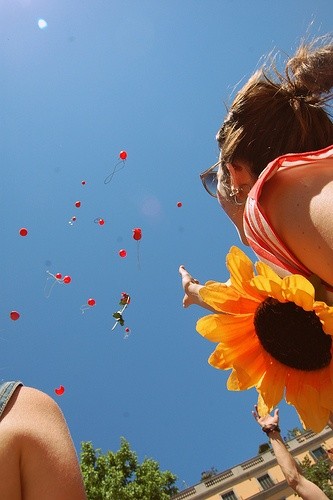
[19,150,183,333]
[10,311,19,320]
[55,385,65,394]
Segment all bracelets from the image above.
[262,424,281,433]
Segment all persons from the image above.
[178,45,333,500]
[0,381,86,500]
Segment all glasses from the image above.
[200,158,232,198]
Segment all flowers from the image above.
[195,245,333,433]
